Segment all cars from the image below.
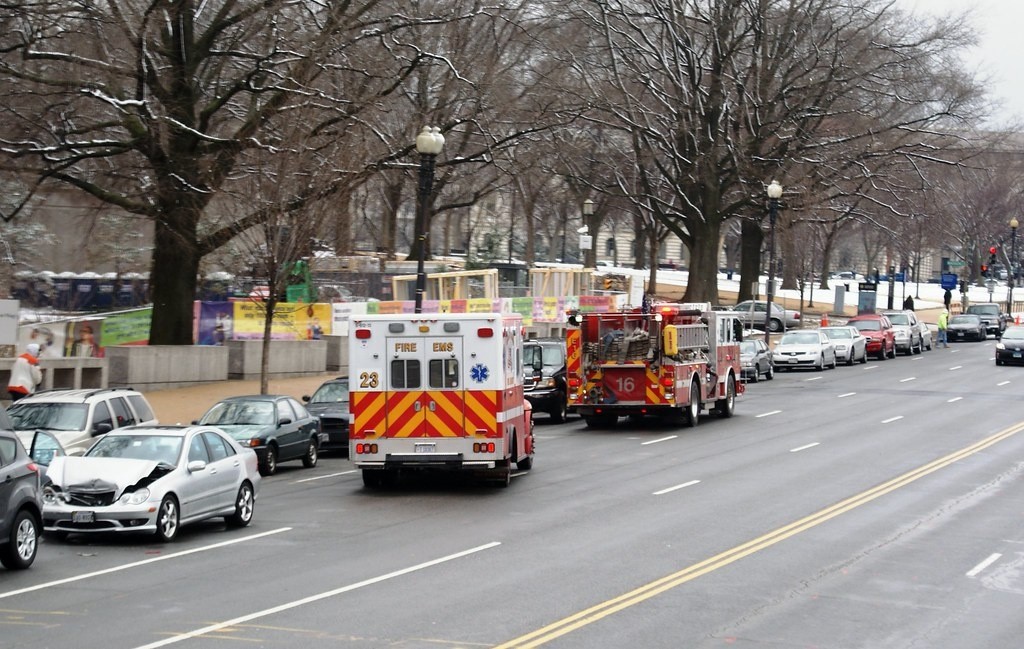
[832,270,905,282]
[772,304,1024,373]
[191,394,324,477]
[730,300,802,332]
[0,402,44,571]
[738,338,776,382]
[301,376,350,450]
[27,425,259,544]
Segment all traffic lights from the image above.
[980,264,988,278]
[990,247,996,265]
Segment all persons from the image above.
[311,317,324,339]
[7,343,42,402]
[905,295,914,311]
[65,326,100,357]
[212,306,233,346]
[935,309,950,348]
[944,288,952,311]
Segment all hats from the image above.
[942,309,948,313]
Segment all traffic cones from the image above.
[820,313,829,327]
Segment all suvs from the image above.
[521,337,585,423]
[6,385,159,470]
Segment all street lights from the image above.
[1007,217,1019,314]
[415,124,444,313]
[765,180,782,346]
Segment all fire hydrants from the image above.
[1014,315,1020,326]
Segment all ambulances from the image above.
[345,311,537,495]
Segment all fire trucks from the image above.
[564,301,747,430]
